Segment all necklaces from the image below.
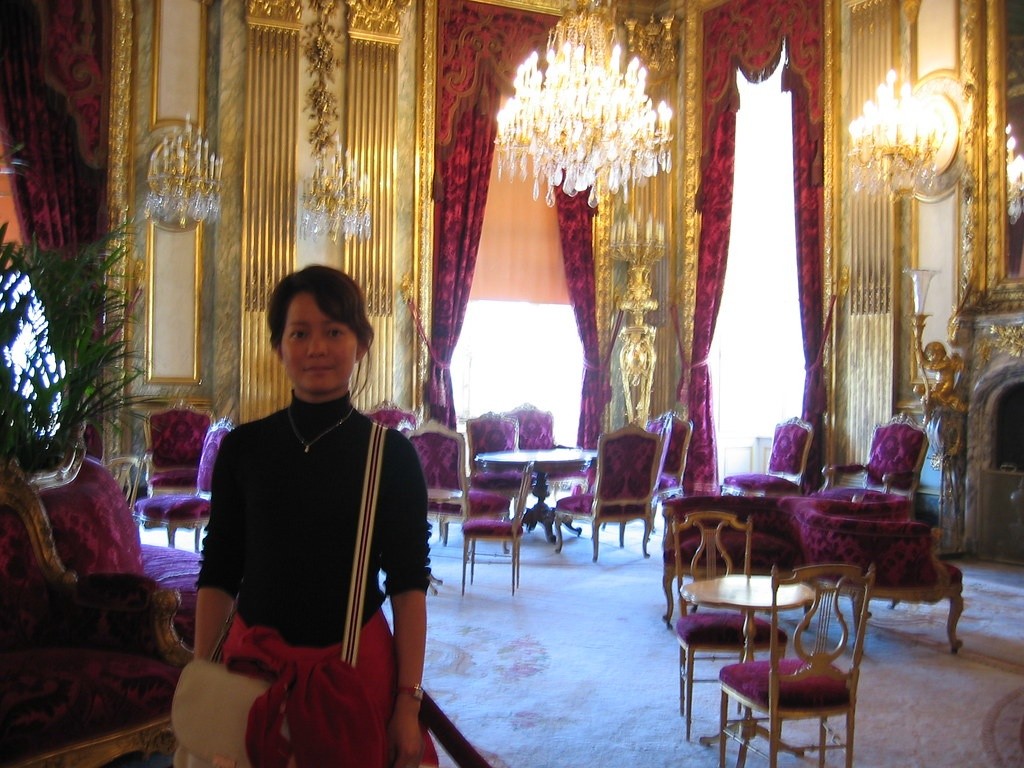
[287,405,354,453]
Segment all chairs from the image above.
[1,402,964,768]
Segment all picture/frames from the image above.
[958,0,1024,313]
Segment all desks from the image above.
[476,450,596,545]
[678,574,813,756]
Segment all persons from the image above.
[193,264,430,767]
[913,342,954,423]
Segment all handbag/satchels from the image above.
[171,658,274,768]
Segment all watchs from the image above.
[401,684,423,700]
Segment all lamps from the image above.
[304,138,373,238]
[501,0,672,209]
[851,69,973,203]
[604,212,668,299]
[143,112,225,224]
